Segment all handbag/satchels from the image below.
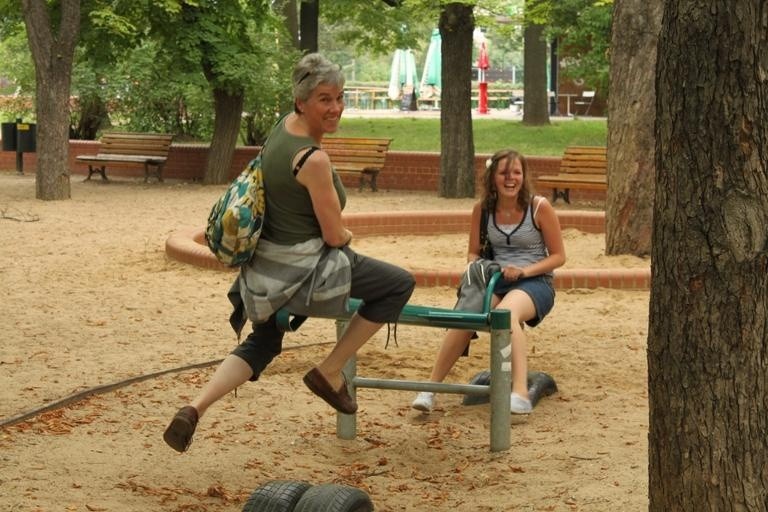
[480,200,495,261]
[204,111,291,267]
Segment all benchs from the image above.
[536,145,609,203]
[76,131,174,184]
[320,137,395,194]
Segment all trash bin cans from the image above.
[1,119,37,153]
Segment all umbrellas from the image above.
[478,40,489,113]
[386,24,420,102]
[417,28,441,109]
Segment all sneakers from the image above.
[412,392,434,415]
[511,392,532,413]
[163,406,198,452]
[303,367,358,414]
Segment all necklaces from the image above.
[498,209,517,220]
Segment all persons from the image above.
[162,51,417,454]
[412,149,565,416]
